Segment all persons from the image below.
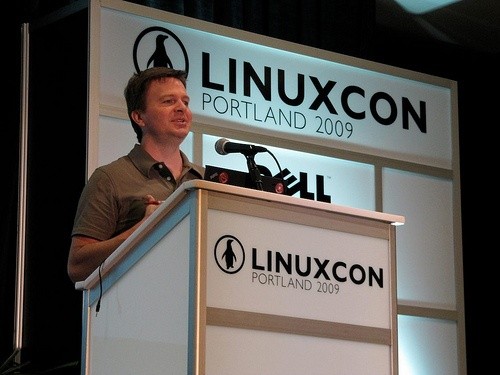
[66,66,209,285]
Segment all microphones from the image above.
[215,138,267,156]
